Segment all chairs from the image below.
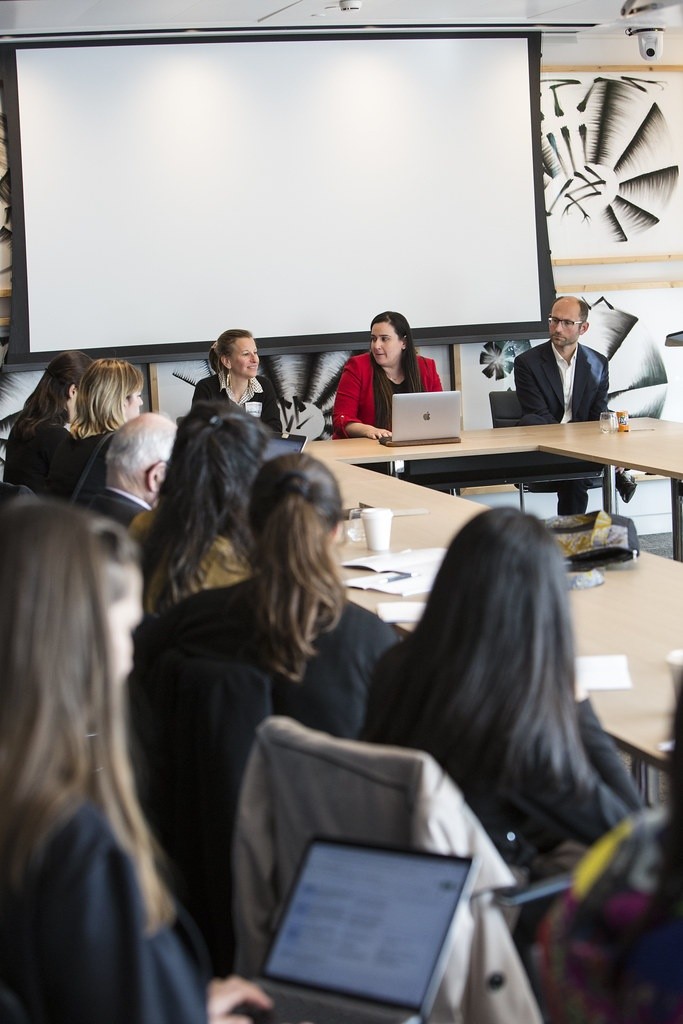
[488,391,618,518]
[230,717,571,1024]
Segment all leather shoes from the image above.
[616,468,637,504]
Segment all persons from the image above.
[332,311,460,497]
[159,451,400,739]
[90,412,178,529]
[3,352,94,496]
[0,501,276,1024]
[190,329,283,433]
[127,399,266,620]
[359,506,645,951]
[514,296,638,516]
[45,358,144,508]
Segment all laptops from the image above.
[391,391,462,441]
[230,833,480,1024]
[263,431,307,462]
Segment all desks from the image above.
[287,417,683,797]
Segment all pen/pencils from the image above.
[624,429,655,432]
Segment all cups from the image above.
[667,650,683,706]
[361,508,393,550]
[348,509,366,541]
[600,413,619,433]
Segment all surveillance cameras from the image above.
[638,32,663,61]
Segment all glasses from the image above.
[546,315,583,329]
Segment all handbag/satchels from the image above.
[536,509,640,592]
